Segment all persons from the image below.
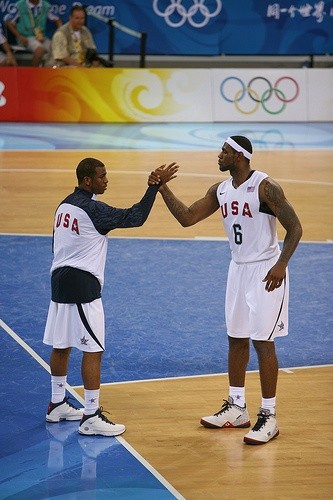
[5,0,61,67]
[0,29,15,66]
[44,157,179,437]
[50,5,103,67]
[147,137,302,446]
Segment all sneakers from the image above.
[78,406,126,437]
[45,396,86,423]
[201,396,251,428]
[244,407,280,444]
